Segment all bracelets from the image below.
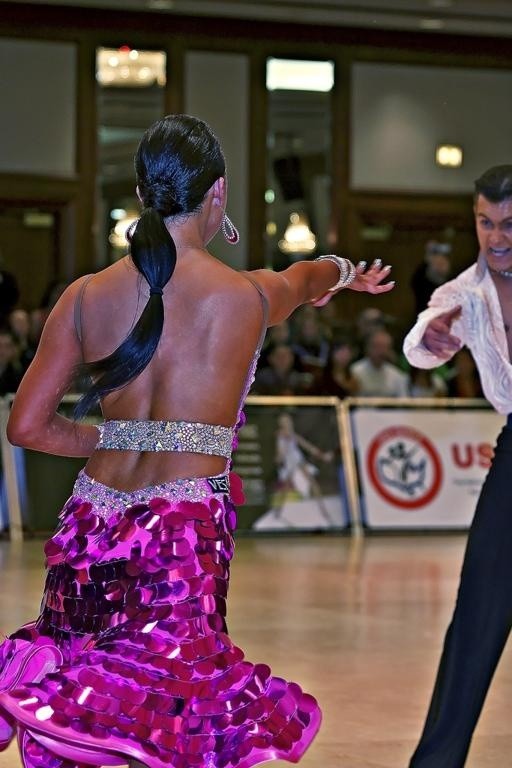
[314,253,358,291]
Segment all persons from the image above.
[250,237,481,400]
[402,164,512,768]
[0,302,49,400]
[270,413,335,525]
[0,112,397,768]
